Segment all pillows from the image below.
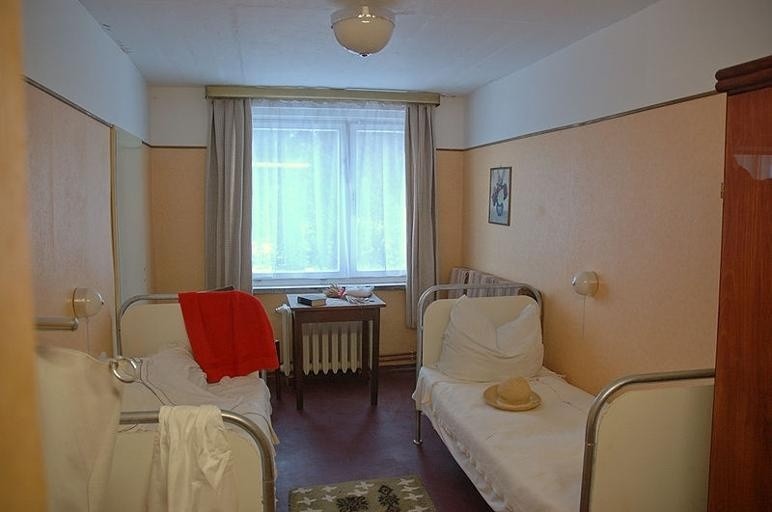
[437,294,544,382]
[106,356,244,433]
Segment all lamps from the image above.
[328,6,396,57]
[570,271,600,342]
[73,287,105,359]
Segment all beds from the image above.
[410,283,715,512]
[36,290,275,511]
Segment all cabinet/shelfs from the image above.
[705,56,771,512]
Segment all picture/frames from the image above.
[488,166,512,226]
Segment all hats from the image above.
[483,376,541,411]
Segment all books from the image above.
[297,293,327,307]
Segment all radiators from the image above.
[274,303,373,376]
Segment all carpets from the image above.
[288,474,437,512]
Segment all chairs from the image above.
[213,285,281,401]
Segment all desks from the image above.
[286,291,387,411]
